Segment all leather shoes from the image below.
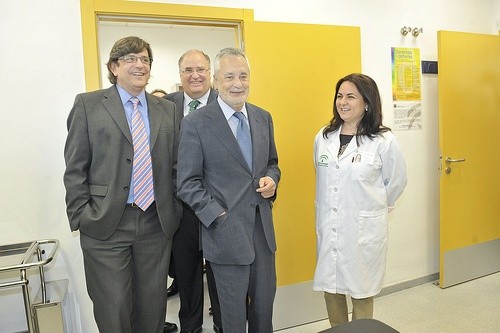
[166,279,179,297]
[163,322,177,332]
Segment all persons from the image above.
[63,35,183,333]
[176,48,281,333]
[159,51,234,333]
[355,154,361,163]
[150,88,215,331]
[313,72,408,328]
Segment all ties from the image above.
[234,111,254,172]
[188,101,201,114]
[129,98,154,213]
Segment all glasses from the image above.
[180,66,210,75]
[116,55,151,65]
